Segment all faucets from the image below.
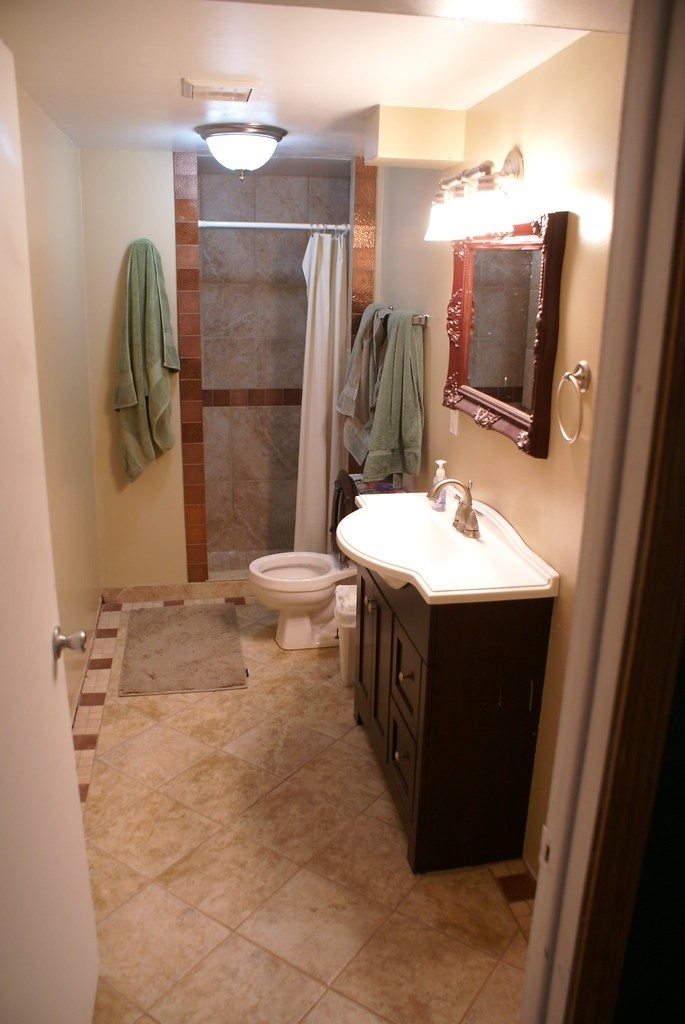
[427,477,481,539]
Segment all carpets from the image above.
[118,603,248,698]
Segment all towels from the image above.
[113,237,181,483]
[335,304,425,491]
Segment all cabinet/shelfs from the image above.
[353,565,554,875]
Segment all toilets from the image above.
[249,469,362,651]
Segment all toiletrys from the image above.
[431,459,446,511]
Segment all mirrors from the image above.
[441,209,567,459]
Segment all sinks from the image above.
[336,494,559,604]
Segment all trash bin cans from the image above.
[333,583,357,686]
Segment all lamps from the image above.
[193,123,287,181]
[423,148,525,242]
